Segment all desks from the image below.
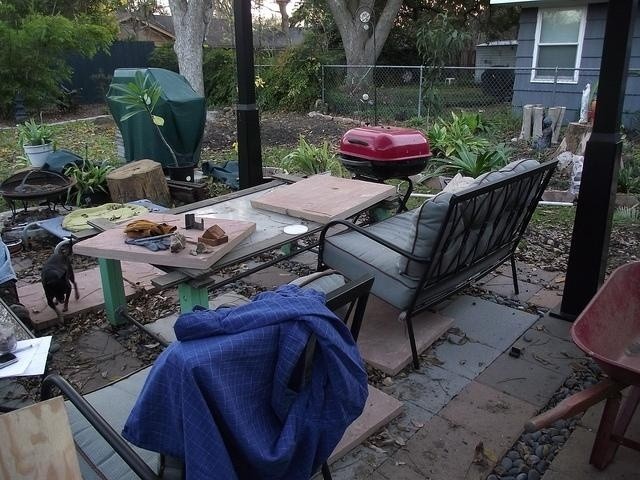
[70,173,395,329]
[0,296,37,412]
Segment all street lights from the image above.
[353,6,378,130]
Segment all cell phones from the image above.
[0,352,18,369]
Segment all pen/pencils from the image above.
[13,345,33,353]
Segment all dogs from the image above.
[40,234,80,324]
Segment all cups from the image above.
[0,322,18,355]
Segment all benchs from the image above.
[316,158,560,371]
[23,199,173,258]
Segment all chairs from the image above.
[40,268,376,479]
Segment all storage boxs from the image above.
[337,126,434,179]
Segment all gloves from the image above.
[124,218,178,252]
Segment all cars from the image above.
[373,58,417,87]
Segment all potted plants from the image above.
[107,70,195,183]
[16,112,55,167]
